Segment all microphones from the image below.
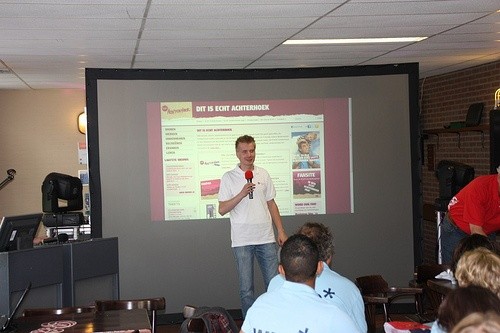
[43,233,68,243]
[245,169,253,199]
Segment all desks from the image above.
[0,244,64,319]
[6,307,151,333]
[65,237,120,307]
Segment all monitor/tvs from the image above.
[0,213,43,252]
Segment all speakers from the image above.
[436,160,474,199]
[41,172,85,212]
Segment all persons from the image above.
[239,235,364,333]
[292,132,320,168]
[217,134,289,321]
[432,164,500,333]
[299,223,368,333]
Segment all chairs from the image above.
[412,263,458,321]
[96,297,165,333]
[355,275,422,333]
